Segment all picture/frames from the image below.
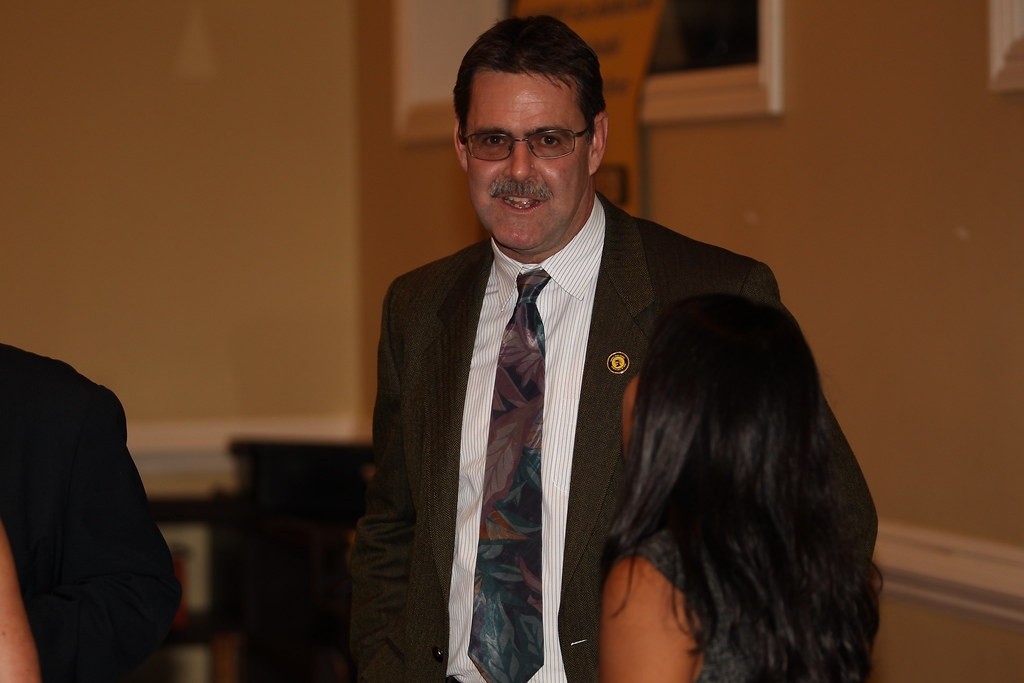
[636,0,785,128]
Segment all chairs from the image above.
[149,440,370,683]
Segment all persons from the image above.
[351,14,879,683]
[0,342,182,683]
[598,292,883,683]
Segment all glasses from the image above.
[458,118,591,162]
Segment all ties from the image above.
[467,269,552,683]
